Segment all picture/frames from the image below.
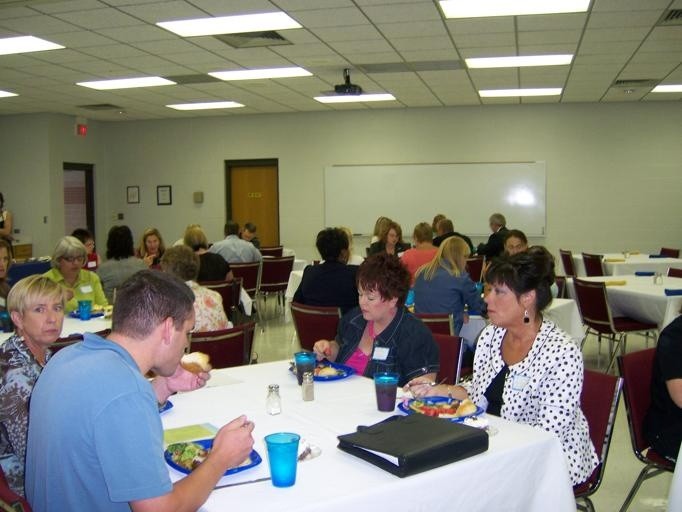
[157,185,172,205]
[126,186,139,204]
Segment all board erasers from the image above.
[353,234,362,238]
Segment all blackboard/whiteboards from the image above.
[323,161,549,239]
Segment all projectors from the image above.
[332,84,362,95]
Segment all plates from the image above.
[164,439,262,476]
[69,311,104,318]
[159,400,173,413]
[467,310,480,315]
[289,359,353,381]
[398,396,484,422]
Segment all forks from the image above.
[298,446,311,460]
[409,377,448,386]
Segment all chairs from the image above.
[0,314,111,341]
[660,247,680,258]
[259,256,295,307]
[260,245,283,256]
[572,370,624,512]
[666,267,682,278]
[432,334,464,386]
[412,313,454,337]
[560,249,576,277]
[187,321,255,369]
[466,256,485,283]
[312,260,321,266]
[227,262,264,333]
[619,347,675,511]
[291,302,341,353]
[198,278,243,309]
[581,253,604,276]
[573,278,658,368]
[555,276,568,299]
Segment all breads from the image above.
[455,398,477,417]
[179,351,211,376]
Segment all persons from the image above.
[292,227,361,316]
[368,216,391,247]
[313,252,439,390]
[208,222,263,263]
[161,245,234,333]
[0,275,65,495]
[370,221,411,259]
[432,219,475,258]
[239,223,261,251]
[311,227,365,266]
[96,227,150,305]
[409,245,598,488]
[0,194,12,239]
[432,214,444,233]
[412,235,487,365]
[24,269,254,512]
[477,213,508,260]
[136,228,166,267]
[72,228,102,271]
[43,235,107,312]
[1,239,22,333]
[644,314,681,459]
[503,230,527,256]
[185,227,234,285]
[402,222,442,288]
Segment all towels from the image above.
[605,280,626,286]
[649,254,668,258]
[665,288,682,296]
[621,249,640,255]
[604,258,626,262]
[635,271,654,276]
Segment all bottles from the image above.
[301,370,314,402]
[654,272,663,286]
[265,383,281,415]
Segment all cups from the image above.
[264,432,300,487]
[373,371,399,411]
[78,300,91,320]
[294,351,316,385]
[0,311,14,332]
[475,282,482,295]
[405,290,415,305]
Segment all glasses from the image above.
[62,254,85,262]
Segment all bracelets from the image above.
[447,385,453,399]
